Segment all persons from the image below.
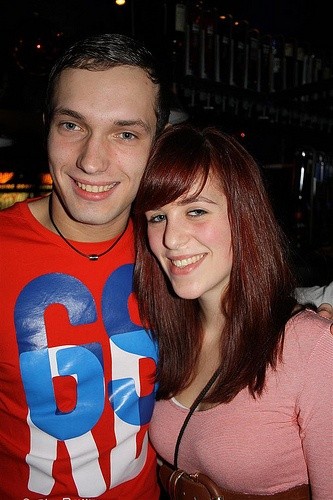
[132,122,333,500]
[0,24,332,500]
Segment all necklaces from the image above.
[47,194,130,261]
